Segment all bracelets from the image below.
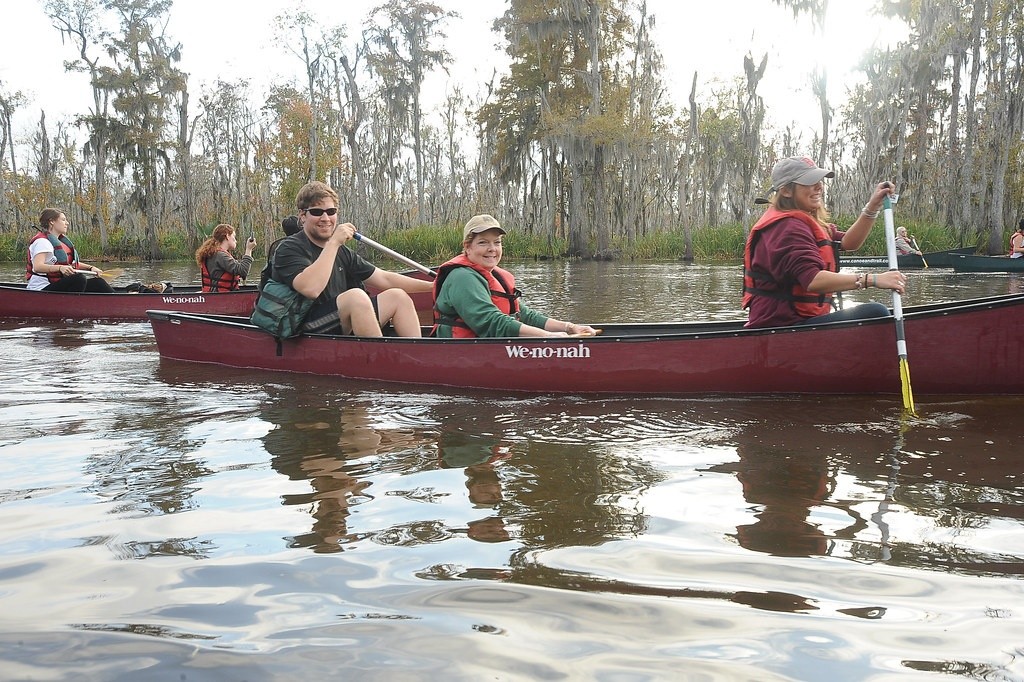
[873,273,876,286]
[49,265,61,272]
[861,202,880,219]
[865,274,868,290]
[854,272,864,290]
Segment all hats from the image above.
[770,156,835,191]
[463,215,507,241]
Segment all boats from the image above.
[949,252,1024,273]
[837,247,977,268]
[0,267,445,318]
[147,294,1023,392]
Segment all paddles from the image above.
[912,238,928,268]
[242,239,255,284]
[74,269,124,281]
[884,181,915,412]
[353,233,438,278]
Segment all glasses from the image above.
[302,208,337,216]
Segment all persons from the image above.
[895,226,922,256]
[195,224,258,293]
[428,214,597,338]
[742,156,908,329]
[1009,218,1024,259]
[250,181,434,342]
[26,208,117,294]
